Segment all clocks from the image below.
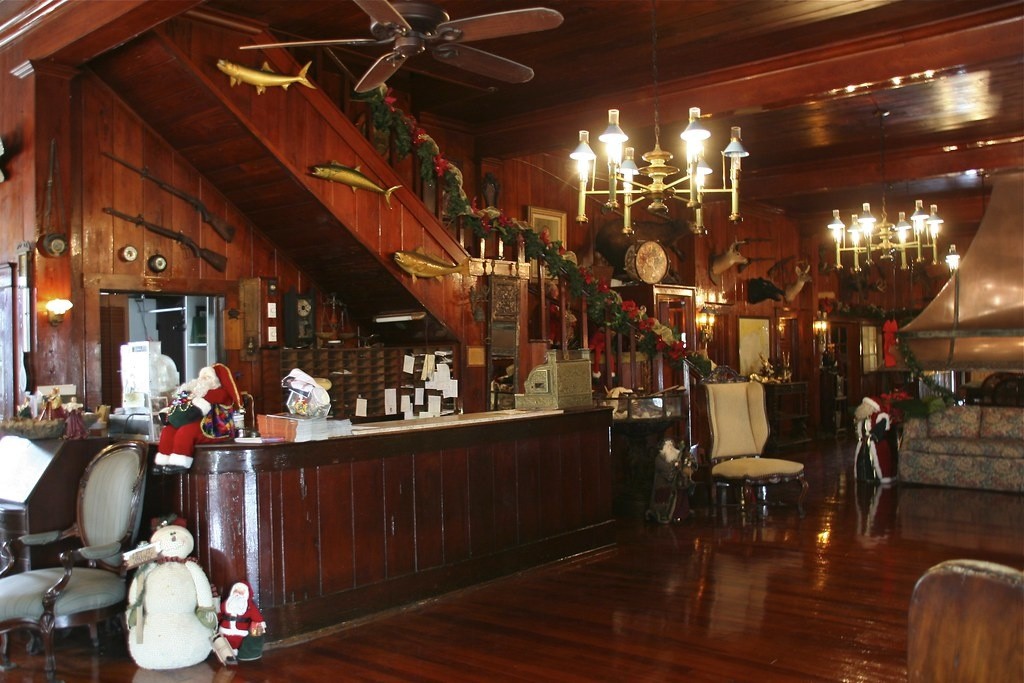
[281,284,317,349]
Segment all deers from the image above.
[785,264,812,302]
[712,235,748,275]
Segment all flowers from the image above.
[353,82,712,375]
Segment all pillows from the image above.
[927,405,982,439]
[979,407,1024,440]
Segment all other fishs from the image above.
[312,159,403,210]
[394,247,472,284]
[216,58,316,95]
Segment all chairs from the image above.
[0,439,148,683]
[699,365,810,527]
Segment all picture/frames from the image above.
[526,205,568,285]
[737,315,772,376]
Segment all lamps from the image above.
[569,0,750,238]
[826,115,961,275]
[699,306,720,343]
[814,312,828,341]
[372,309,427,323]
[45,297,73,328]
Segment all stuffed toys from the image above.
[124,524,219,670]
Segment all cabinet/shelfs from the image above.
[261,342,459,416]
[766,381,813,455]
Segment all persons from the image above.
[215,579,268,662]
[854,393,898,482]
[17,387,89,440]
[151,363,243,477]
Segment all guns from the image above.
[100,150,236,244]
[102,207,228,273]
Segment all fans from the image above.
[239,0,565,93]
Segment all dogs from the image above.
[748,277,785,304]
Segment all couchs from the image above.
[896,405,1024,492]
